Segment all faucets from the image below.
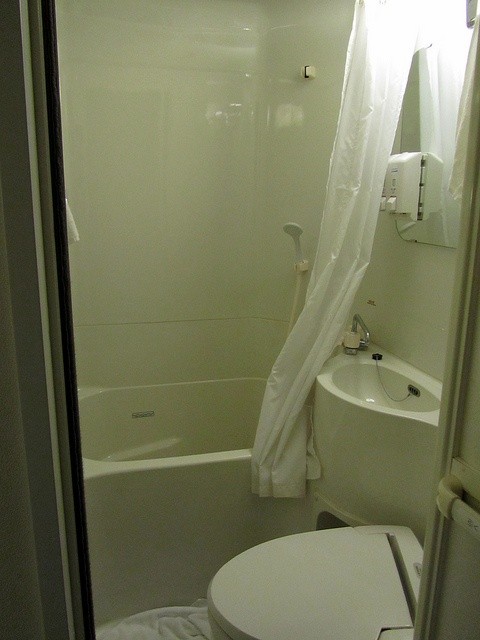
[344,313,371,354]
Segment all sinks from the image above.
[331,362,442,412]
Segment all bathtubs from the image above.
[80,379,294,625]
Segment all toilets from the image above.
[206,523,424,640]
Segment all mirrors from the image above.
[395,42,471,249]
